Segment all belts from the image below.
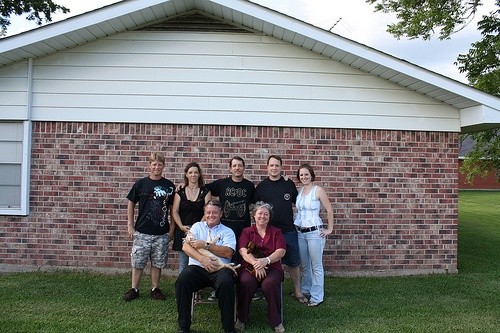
[296,225,324,233]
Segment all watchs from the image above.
[204,242,211,250]
[265,257,272,266]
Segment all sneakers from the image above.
[235,318,245,333]
[251,293,260,300]
[261,292,266,300]
[194,290,205,302]
[177,325,190,333]
[207,291,218,301]
[150,288,165,299]
[274,323,285,333]
[224,326,241,333]
[122,288,139,301]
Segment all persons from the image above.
[284,164,333,306]
[175,200,236,333]
[248,155,308,304]
[236,200,286,333]
[172,161,211,275]
[123,153,175,302]
[172,156,260,300]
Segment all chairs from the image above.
[191,258,284,324]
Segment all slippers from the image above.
[295,295,308,304]
[289,291,296,296]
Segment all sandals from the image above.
[308,298,323,306]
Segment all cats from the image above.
[182,231,241,276]
[242,242,274,281]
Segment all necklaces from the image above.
[187,185,200,196]
